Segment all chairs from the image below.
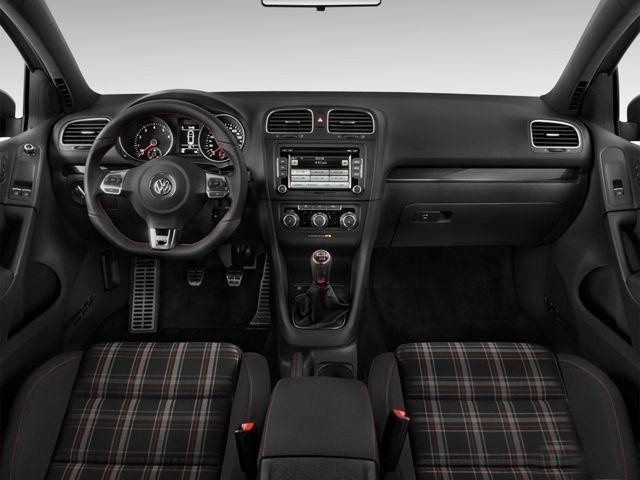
[365,340,634,479]
[4,341,271,480]
